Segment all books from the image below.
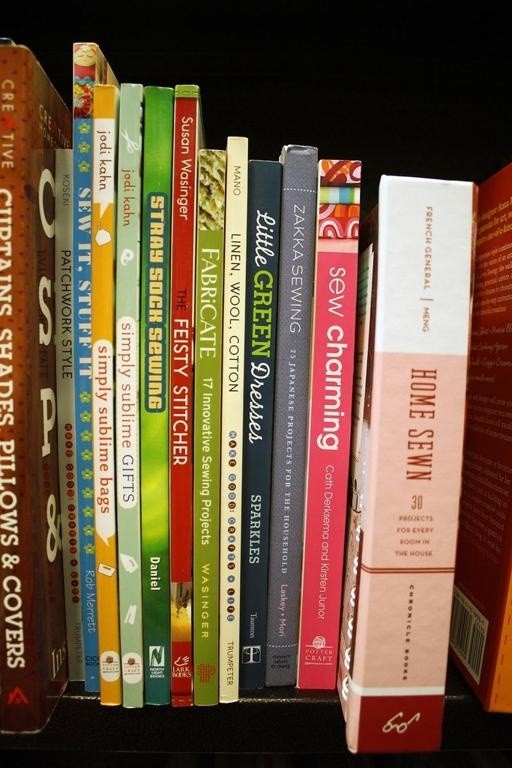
[0,36,512,756]
